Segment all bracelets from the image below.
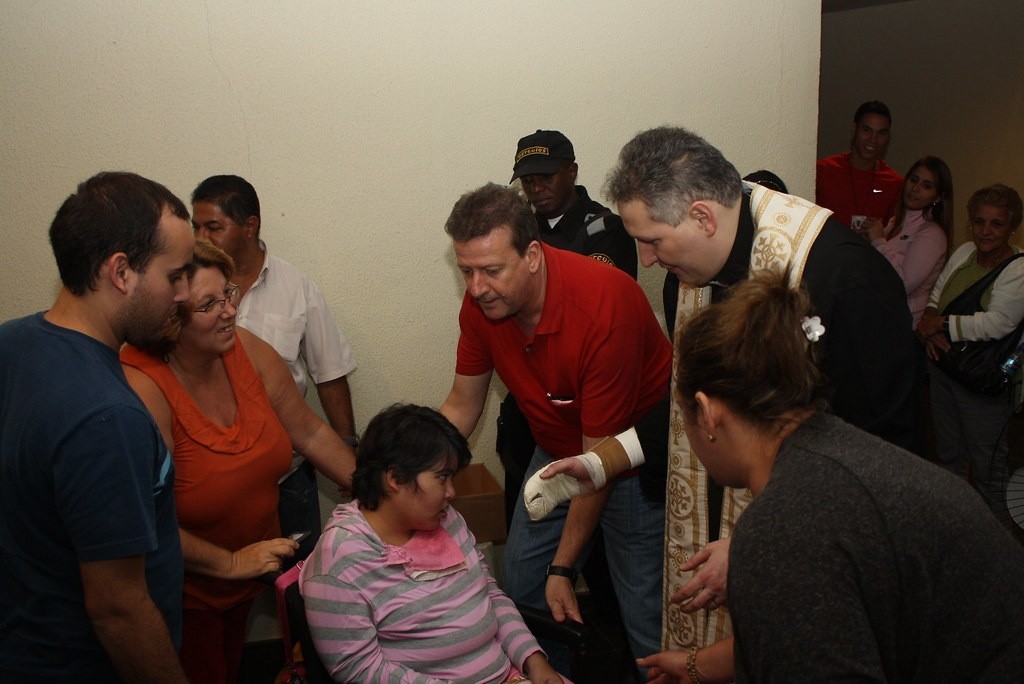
[339,434,360,448]
[687,647,700,683]
[547,565,573,580]
[943,316,949,333]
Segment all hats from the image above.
[510,130,575,184]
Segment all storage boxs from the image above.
[448,460,506,545]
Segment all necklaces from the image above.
[977,249,1008,268]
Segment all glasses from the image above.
[191,283,240,318]
[757,180,782,190]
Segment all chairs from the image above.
[276,454,325,679]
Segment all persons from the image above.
[190,175,360,454]
[299,404,583,684]
[444,102,1024,684]
[121,239,362,683]
[0,173,195,684]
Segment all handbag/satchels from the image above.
[925,253,1024,403]
